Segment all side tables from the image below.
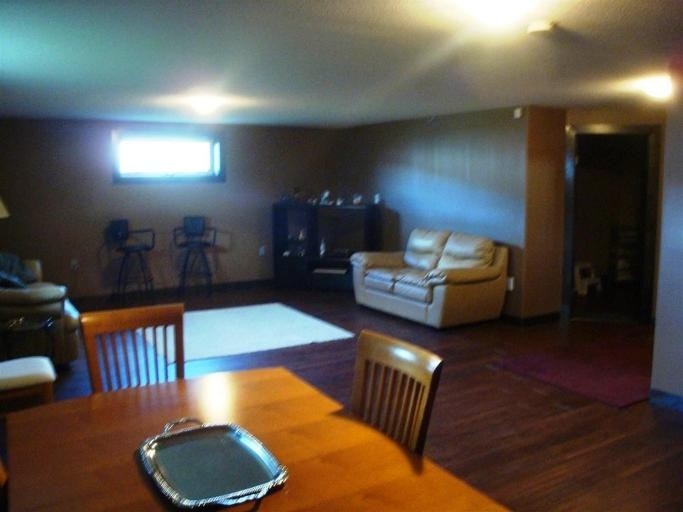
[0,317,63,380]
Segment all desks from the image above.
[0,364,514,512]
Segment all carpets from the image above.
[491,324,654,408]
[135,302,356,367]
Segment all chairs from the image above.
[109,219,156,307]
[172,215,217,300]
[79,302,186,392]
[347,328,444,456]
[0,355,58,421]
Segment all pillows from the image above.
[0,253,37,288]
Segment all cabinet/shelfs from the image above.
[271,202,382,292]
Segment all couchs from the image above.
[0,260,83,375]
[349,227,510,330]
